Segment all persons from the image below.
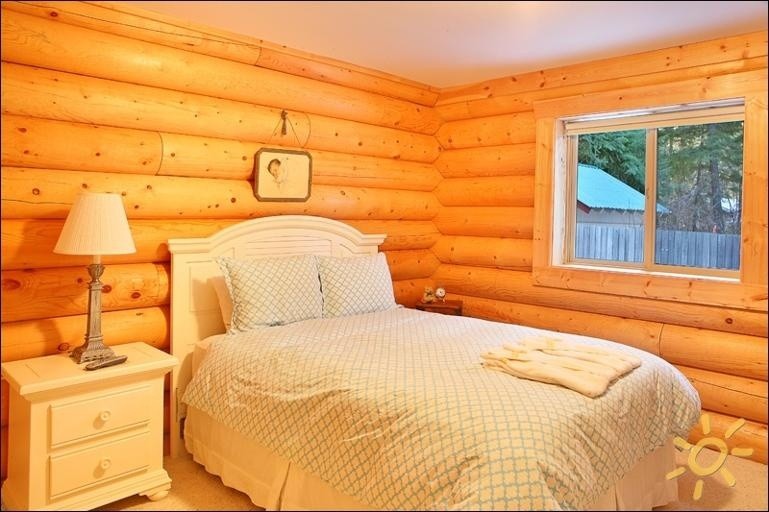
[267,159,293,189]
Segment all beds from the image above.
[167,215,702,511]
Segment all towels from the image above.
[479,337,642,399]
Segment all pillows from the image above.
[314,250,402,318]
[212,276,234,331]
[216,253,324,334]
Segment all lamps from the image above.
[53,192,137,363]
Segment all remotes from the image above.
[86,354,129,370]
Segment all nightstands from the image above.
[0,340,179,511]
[415,299,464,315]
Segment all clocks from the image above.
[435,288,446,303]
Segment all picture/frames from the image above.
[253,148,312,202]
